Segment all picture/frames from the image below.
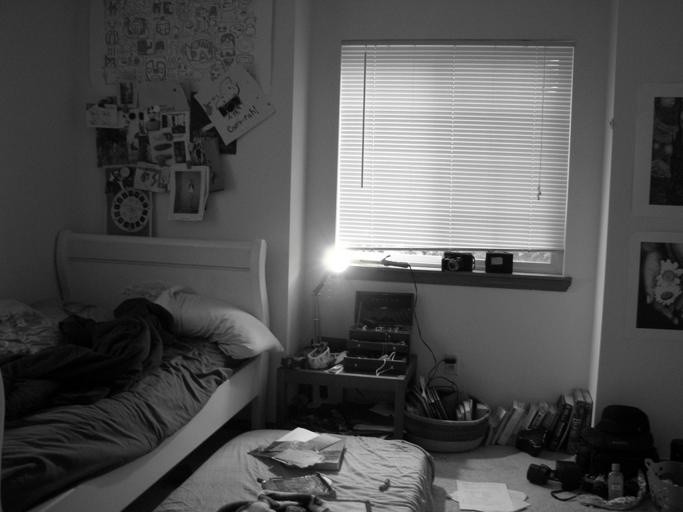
[623,84,683,343]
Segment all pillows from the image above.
[155,283,285,362]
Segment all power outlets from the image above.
[442,353,458,375]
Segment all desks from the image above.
[276,347,417,440]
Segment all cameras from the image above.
[441,252,473,272]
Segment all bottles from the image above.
[607,463,624,500]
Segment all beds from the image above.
[0,229,270,511]
[149,429,436,511]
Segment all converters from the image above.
[445,357,456,363]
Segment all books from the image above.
[486,389,593,447]
[309,436,345,470]
[455,398,473,421]
[415,377,447,419]
